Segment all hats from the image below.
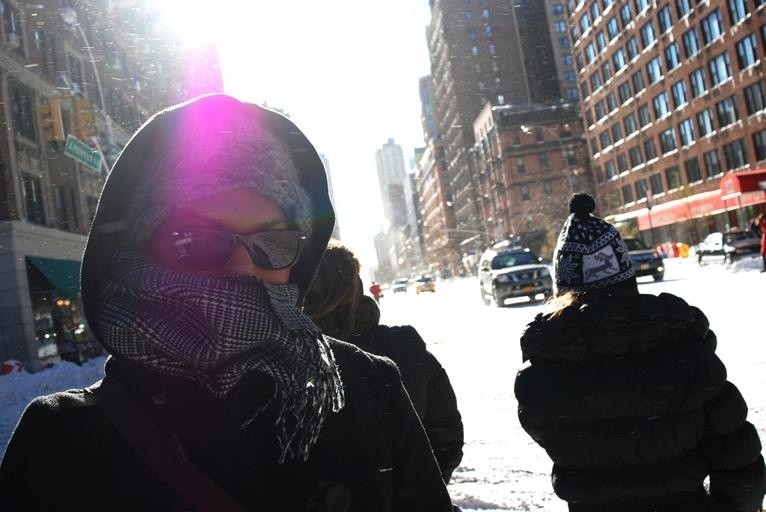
[552,212,639,298]
[124,115,315,262]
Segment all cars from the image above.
[697,230,761,264]
[392,279,408,293]
[622,237,665,282]
[478,246,554,307]
[415,274,436,294]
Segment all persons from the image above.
[513,192,766,512]
[369,281,382,305]
[0,91,460,510]
[751,211,766,274]
[300,234,464,488]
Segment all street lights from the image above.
[60,6,114,169]
[521,126,574,195]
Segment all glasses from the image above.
[157,224,310,272]
